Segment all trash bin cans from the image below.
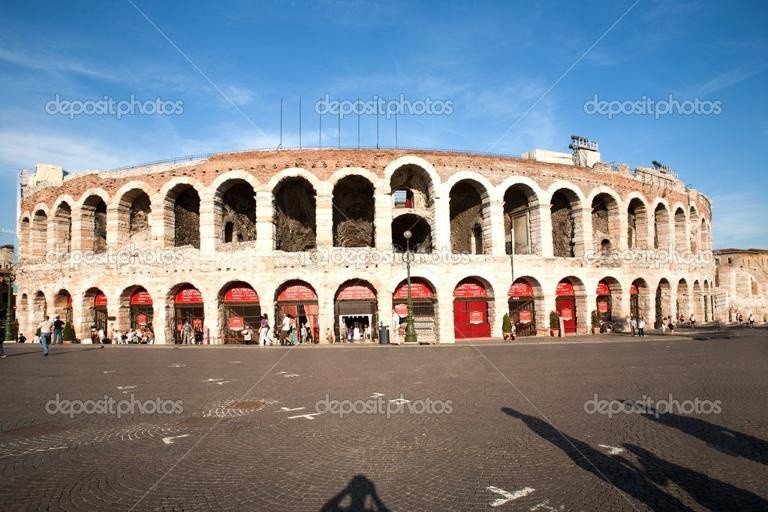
[379,326,389,345]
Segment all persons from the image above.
[0,316,7,358]
[748,313,755,328]
[325,319,369,345]
[52,317,64,345]
[510,323,517,340]
[97,323,154,344]
[179,320,202,346]
[739,314,743,328]
[630,314,698,337]
[240,312,312,345]
[38,315,54,356]
[18,334,26,343]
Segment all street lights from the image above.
[400,230,419,341]
[3,273,18,343]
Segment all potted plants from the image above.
[62,321,74,344]
[549,309,561,337]
[591,310,602,335]
[501,312,513,342]
